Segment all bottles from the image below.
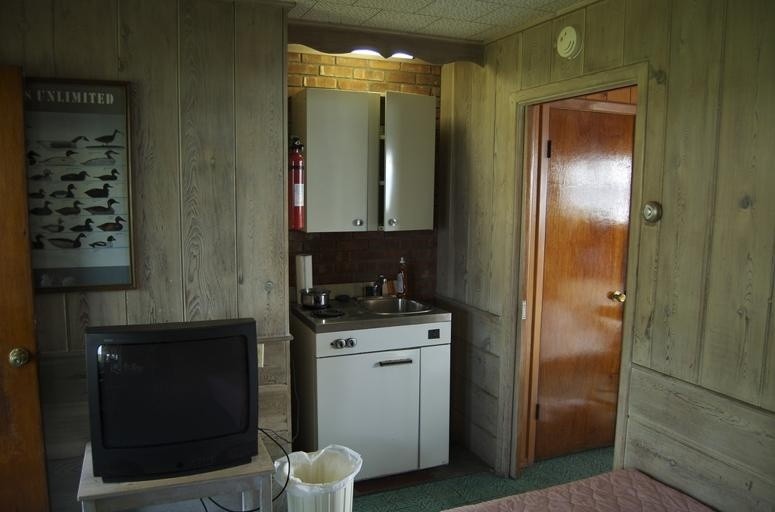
[395,256,411,298]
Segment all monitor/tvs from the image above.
[83,317,259,482]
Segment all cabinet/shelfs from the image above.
[289,87,437,237]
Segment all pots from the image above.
[299,287,331,308]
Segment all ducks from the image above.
[26,130,127,250]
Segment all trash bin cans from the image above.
[283,450,362,512]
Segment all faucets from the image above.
[372,276,387,297]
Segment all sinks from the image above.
[356,297,435,316]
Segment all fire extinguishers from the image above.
[289,136,305,231]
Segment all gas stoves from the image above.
[292,296,353,326]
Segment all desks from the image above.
[75,435,276,511]
